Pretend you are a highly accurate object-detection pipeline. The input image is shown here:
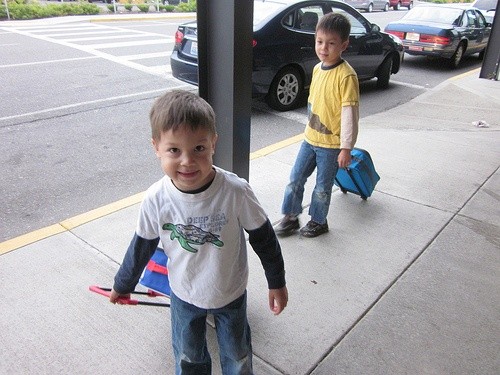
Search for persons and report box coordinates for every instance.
[110,91,289,375]
[273,13,360,238]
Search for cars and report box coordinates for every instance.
[384,2,493,69]
[472,0,497,26]
[345,0,390,13]
[170,0,404,110]
[389,0,414,10]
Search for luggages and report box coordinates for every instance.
[89,248,170,307]
[333,146,380,200]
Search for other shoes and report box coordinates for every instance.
[298,219,328,238]
[273,215,300,235]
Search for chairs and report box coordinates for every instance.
[300,11,318,32]
